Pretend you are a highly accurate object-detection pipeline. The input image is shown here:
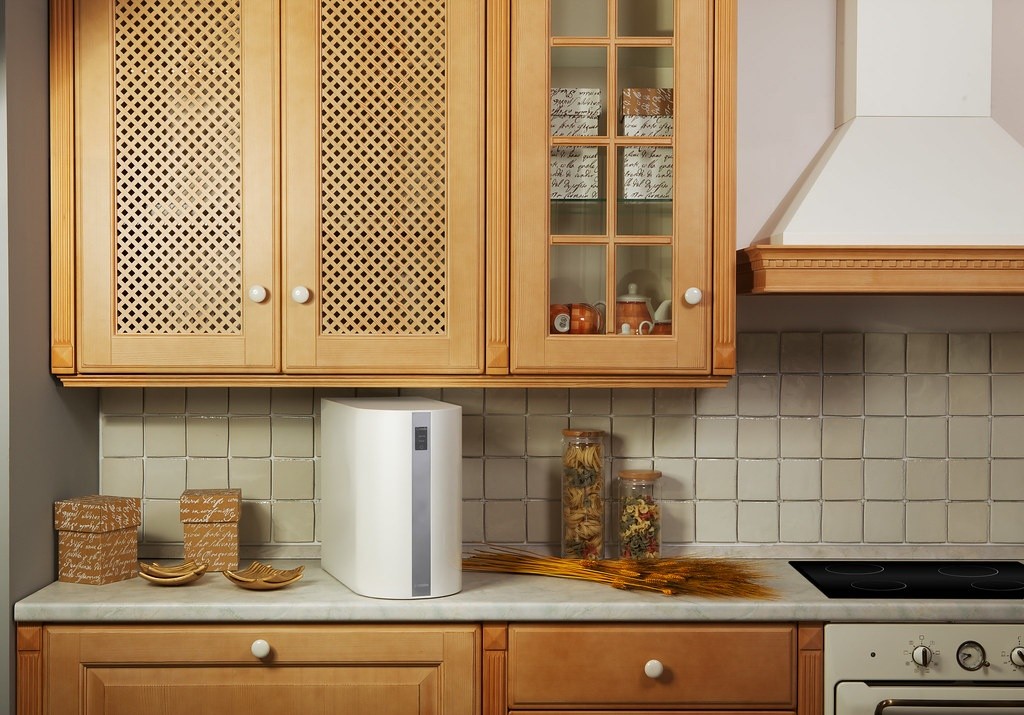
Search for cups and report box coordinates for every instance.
[638,319,672,335]
[551,300,606,334]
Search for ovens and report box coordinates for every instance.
[824,624,1024,715]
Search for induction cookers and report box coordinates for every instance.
[788,560,1024,599]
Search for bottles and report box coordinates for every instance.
[615,469,662,560]
[561,427,606,561]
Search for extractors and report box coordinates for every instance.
[736,0,1023,296]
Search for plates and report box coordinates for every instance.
[222,561,306,590]
[138,557,209,586]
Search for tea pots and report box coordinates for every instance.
[591,283,672,335]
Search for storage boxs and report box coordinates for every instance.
[620,84,676,117]
[549,116,599,199]
[53,493,142,535]
[623,118,674,203]
[185,523,238,575]
[59,532,138,587]
[179,487,241,522]
[547,85,600,115]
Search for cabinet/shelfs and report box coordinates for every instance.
[15,623,824,715]
[51,0,739,389]
